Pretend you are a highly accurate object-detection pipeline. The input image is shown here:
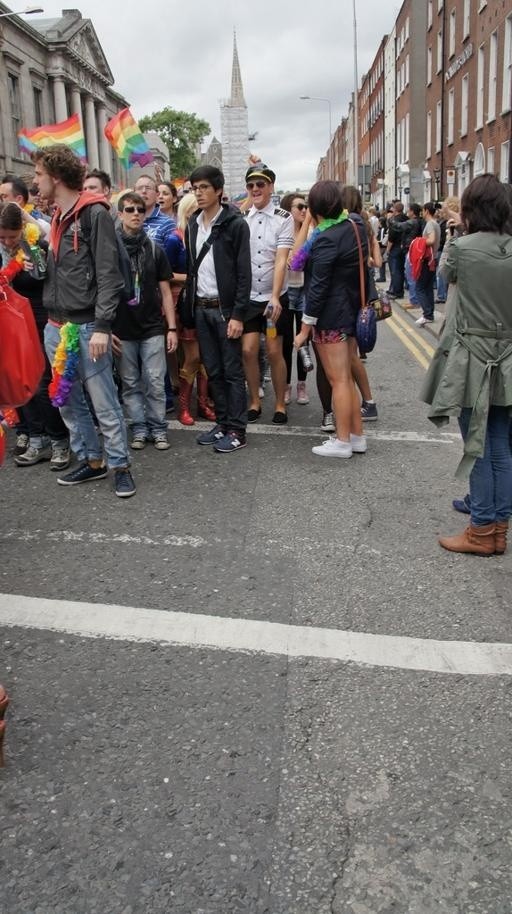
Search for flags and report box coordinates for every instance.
[103,105,154,170]
[17,109,86,167]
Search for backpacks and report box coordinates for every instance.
[78,204,135,302]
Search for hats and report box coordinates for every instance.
[244,162,275,183]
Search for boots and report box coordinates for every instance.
[196,372,217,422]
[439,522,496,558]
[496,517,508,556]
[177,375,196,425]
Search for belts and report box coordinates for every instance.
[195,299,220,310]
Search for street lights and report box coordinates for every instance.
[0,6,44,22]
[301,96,333,181]
[433,167,441,204]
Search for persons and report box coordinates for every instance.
[1,143,385,496]
[362,193,463,326]
[417,174,512,557]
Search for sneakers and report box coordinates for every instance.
[296,382,310,405]
[195,426,227,444]
[114,470,137,498]
[154,433,170,450]
[131,436,147,450]
[272,408,288,424]
[248,375,271,422]
[311,401,377,459]
[416,317,433,324]
[58,462,108,486]
[14,433,70,471]
[214,432,247,452]
[285,384,293,404]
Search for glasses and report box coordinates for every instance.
[191,184,211,192]
[291,204,307,210]
[124,207,145,213]
[246,182,267,189]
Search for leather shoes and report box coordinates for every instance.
[454,500,471,514]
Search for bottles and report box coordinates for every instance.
[28,242,46,278]
[298,341,314,373]
[265,305,278,339]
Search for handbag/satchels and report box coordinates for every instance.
[369,296,393,319]
[174,283,196,330]
[0,284,47,407]
[357,306,378,353]
